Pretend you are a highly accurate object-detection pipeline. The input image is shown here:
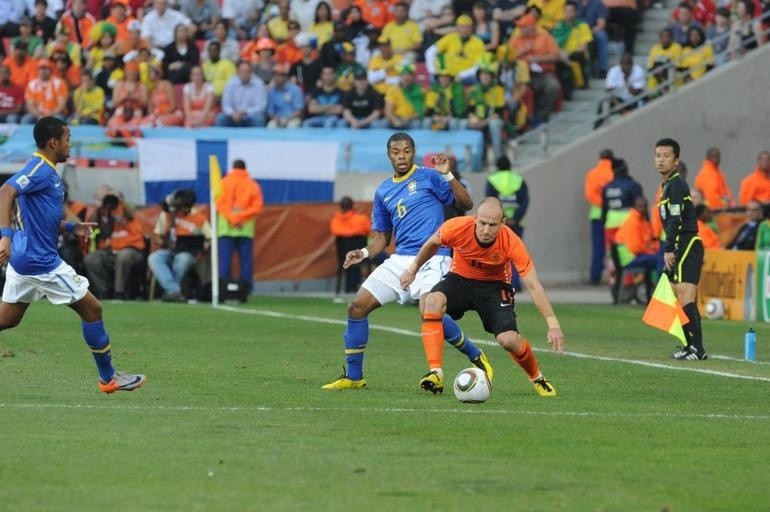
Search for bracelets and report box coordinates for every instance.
[2,226,14,239]
[546,316,561,329]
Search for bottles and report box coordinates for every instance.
[743,327,758,363]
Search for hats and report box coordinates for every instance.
[434,15,537,81]
[333,21,419,73]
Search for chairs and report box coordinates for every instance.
[606,239,653,307]
[334,235,367,296]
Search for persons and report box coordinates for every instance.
[654,139,709,360]
[401,196,564,396]
[59,186,212,302]
[1,116,148,394]
[329,154,529,303]
[0,0,770,141]
[214,160,263,305]
[320,132,493,390]
[584,137,770,303]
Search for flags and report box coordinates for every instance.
[642,274,690,346]
[210,156,223,198]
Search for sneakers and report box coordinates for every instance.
[472,348,493,380]
[680,344,707,360]
[529,371,557,396]
[322,375,367,389]
[671,345,688,359]
[98,373,146,394]
[419,370,443,394]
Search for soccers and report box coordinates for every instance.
[706,299,728,319]
[453,367,492,402]
